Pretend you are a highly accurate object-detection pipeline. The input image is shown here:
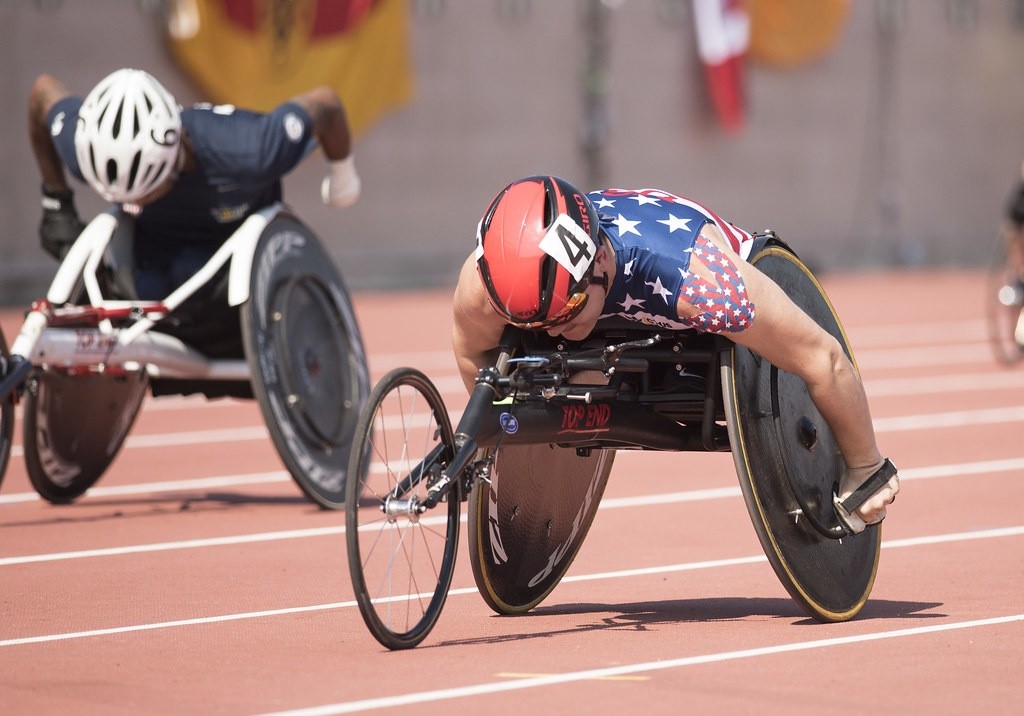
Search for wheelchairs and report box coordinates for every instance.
[344,229,896,650]
[0,203,373,510]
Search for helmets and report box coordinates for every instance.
[74,69,181,204]
[473,175,601,331]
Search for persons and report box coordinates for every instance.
[25,67,366,340]
[454,176,902,527]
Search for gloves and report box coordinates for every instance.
[320,155,361,208]
[34,187,86,259]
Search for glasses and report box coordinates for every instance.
[487,292,590,332]
